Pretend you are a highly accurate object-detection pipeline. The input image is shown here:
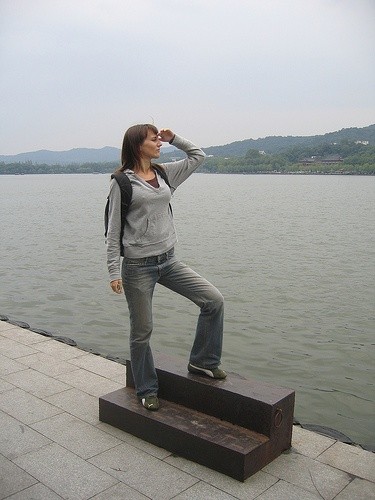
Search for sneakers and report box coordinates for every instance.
[187,362,228,380]
[141,395,160,410]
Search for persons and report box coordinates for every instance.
[105,124,228,411]
